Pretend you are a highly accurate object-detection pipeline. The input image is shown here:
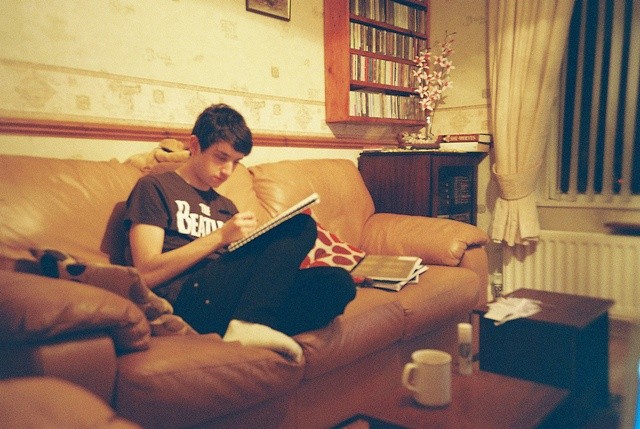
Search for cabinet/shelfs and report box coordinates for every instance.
[471,287,614,407]
[323,0,430,126]
[358,152,488,227]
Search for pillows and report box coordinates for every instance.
[27,245,199,333]
[299,207,369,273]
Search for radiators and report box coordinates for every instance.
[501,229,639,324]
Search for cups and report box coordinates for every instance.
[401,348,452,407]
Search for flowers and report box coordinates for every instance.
[412,29,458,125]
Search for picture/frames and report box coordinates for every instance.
[245,0,292,22]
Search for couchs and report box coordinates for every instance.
[1,153,491,429]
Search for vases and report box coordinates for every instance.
[426,114,434,140]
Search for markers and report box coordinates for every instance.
[458,323,472,375]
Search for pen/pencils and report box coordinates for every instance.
[218,209,234,217]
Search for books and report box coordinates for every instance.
[436,134,493,142]
[228,192,320,252]
[326,2,429,122]
[436,144,489,154]
[352,253,429,292]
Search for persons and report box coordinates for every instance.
[124,103,356,363]
